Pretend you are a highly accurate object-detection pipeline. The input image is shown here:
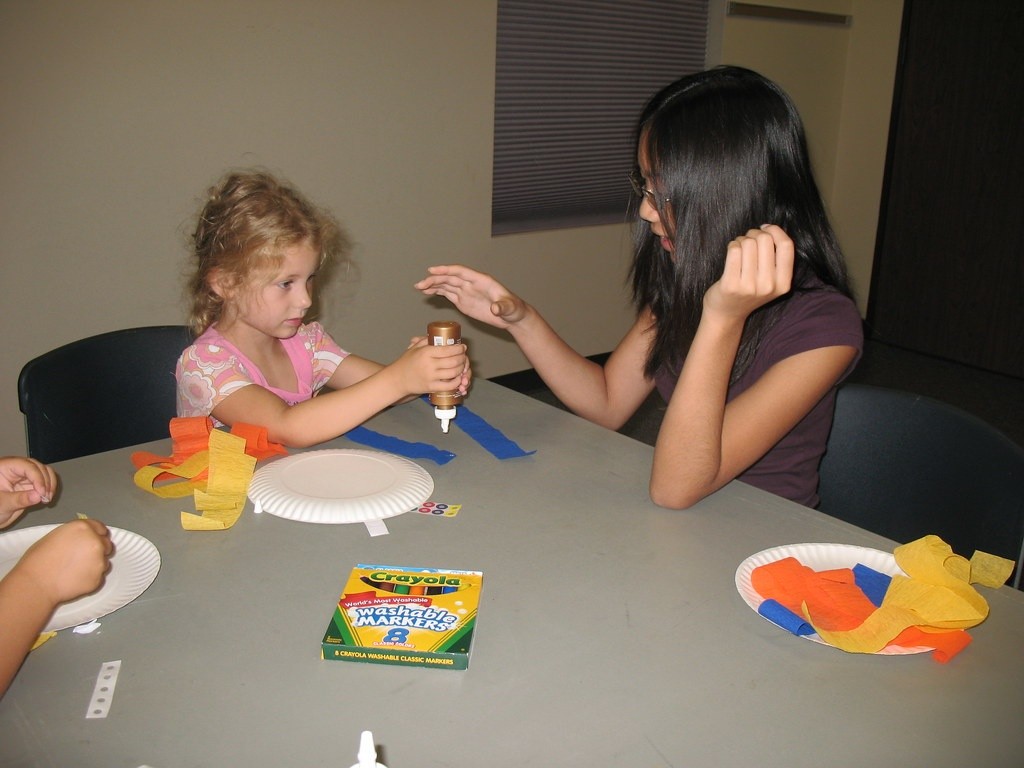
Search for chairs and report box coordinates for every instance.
[18,325,200,463]
[816,383,1023,591]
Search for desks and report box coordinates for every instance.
[0,375,1024,768]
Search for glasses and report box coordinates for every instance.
[627,170,671,211]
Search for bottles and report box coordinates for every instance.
[428,322,461,433]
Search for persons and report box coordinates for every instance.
[0,454,115,703]
[175,170,471,448]
[414,65,865,511]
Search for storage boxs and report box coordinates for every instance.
[320,563,484,670]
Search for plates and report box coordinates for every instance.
[0,523,161,634]
[248,449,435,524]
[735,543,935,655]
